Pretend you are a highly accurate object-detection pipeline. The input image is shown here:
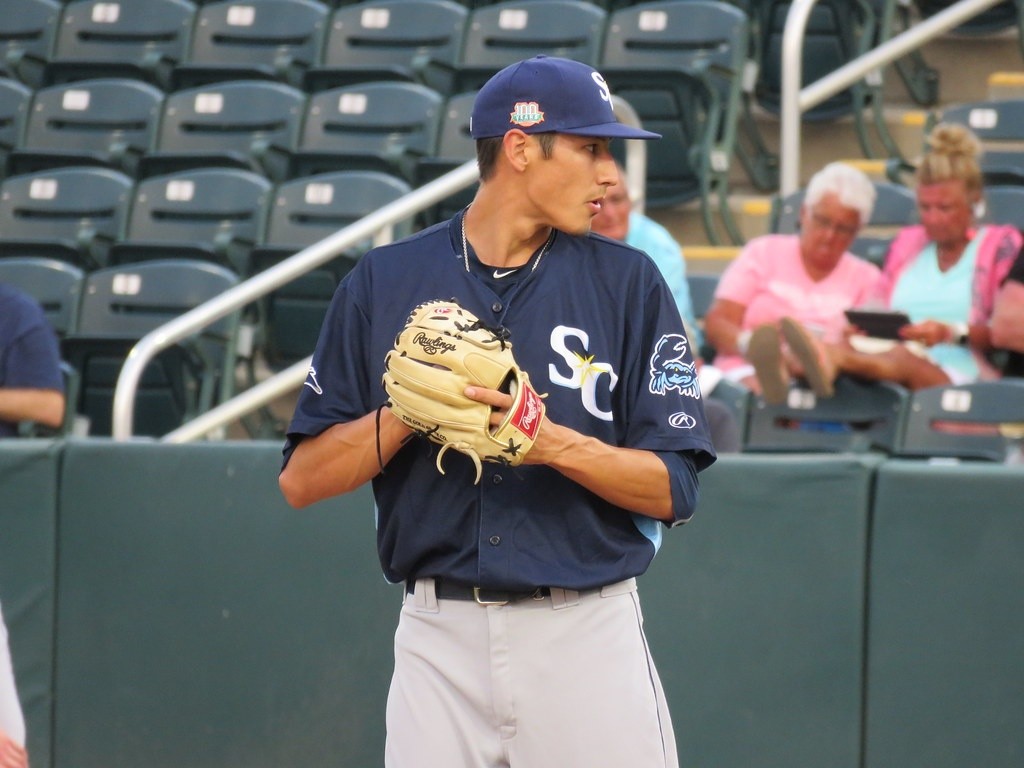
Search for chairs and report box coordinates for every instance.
[0,0,1022,765]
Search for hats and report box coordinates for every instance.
[469,54,663,141]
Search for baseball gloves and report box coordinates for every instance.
[372,295,552,487]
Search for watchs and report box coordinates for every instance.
[955,322,970,346]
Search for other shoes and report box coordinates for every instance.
[778,316,834,401]
[747,324,790,405]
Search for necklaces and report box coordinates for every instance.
[462,214,550,272]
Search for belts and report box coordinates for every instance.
[406,574,551,607]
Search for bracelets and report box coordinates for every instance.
[736,329,753,356]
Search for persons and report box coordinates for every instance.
[0,290,67,768]
[278,52,717,768]
[706,122,1024,439]
[591,162,707,373]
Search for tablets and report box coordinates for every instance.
[842,309,910,340]
